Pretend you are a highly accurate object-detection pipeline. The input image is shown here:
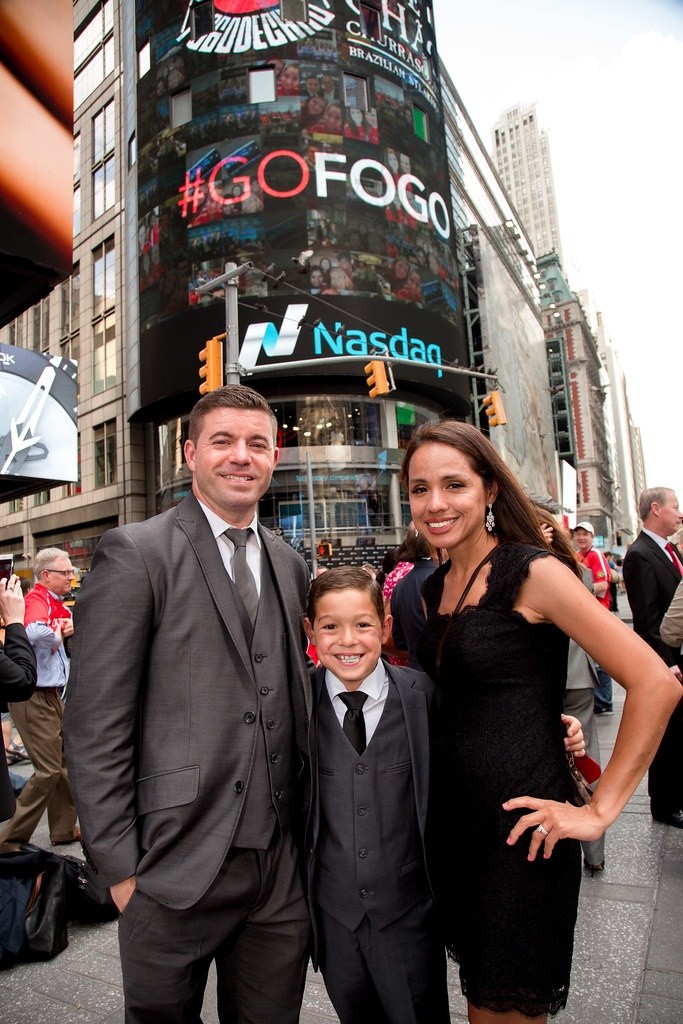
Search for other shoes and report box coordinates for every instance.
[594,704,612,714]
[52,826,81,845]
[653,810,683,829]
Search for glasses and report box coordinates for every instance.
[48,569,74,576]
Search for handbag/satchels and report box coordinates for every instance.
[0,842,119,971]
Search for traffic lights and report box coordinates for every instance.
[199,340,223,396]
[483,391,507,427]
[319,543,332,556]
[364,360,388,398]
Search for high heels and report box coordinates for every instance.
[584,858,604,877]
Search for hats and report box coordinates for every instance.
[573,522,594,535]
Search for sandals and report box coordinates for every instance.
[6,741,30,766]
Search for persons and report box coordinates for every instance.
[137,27,458,312]
[0,547,81,855]
[317,522,622,878]
[401,420,683,1024]
[305,486,586,1024]
[60,384,312,1024]
[622,487,683,828]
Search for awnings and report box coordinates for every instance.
[0,342,78,504]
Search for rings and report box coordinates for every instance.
[537,825,548,835]
[7,586,14,592]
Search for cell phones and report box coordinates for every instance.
[0,553,14,590]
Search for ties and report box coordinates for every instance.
[338,691,368,756]
[664,542,682,577]
[224,530,259,627]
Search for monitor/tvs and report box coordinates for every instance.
[420,280,443,303]
